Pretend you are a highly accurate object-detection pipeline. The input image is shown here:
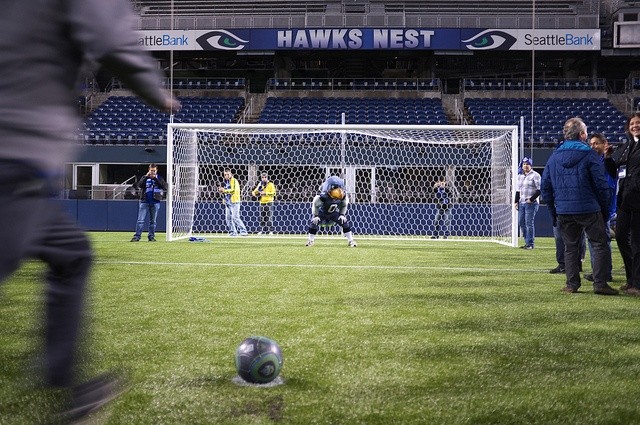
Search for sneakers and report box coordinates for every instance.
[148,238,156,242]
[130,238,139,242]
[584,274,612,282]
[348,240,357,247]
[628,288,640,294]
[620,283,631,290]
[594,284,619,295]
[520,243,534,249]
[305,240,313,246]
[561,286,578,293]
[550,264,565,273]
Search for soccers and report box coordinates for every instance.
[235,337,282,384]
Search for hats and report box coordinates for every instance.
[518,156,533,175]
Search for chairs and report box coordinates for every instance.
[60,93,247,146]
[249,96,452,142]
[465,95,635,148]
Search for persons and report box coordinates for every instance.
[540,118,619,294]
[549,217,586,274]
[0,0,181,419]
[251,169,276,235]
[430,177,451,238]
[305,175,357,247]
[585,134,617,280]
[604,115,640,294]
[129,163,167,242]
[218,168,247,235]
[514,157,542,249]
